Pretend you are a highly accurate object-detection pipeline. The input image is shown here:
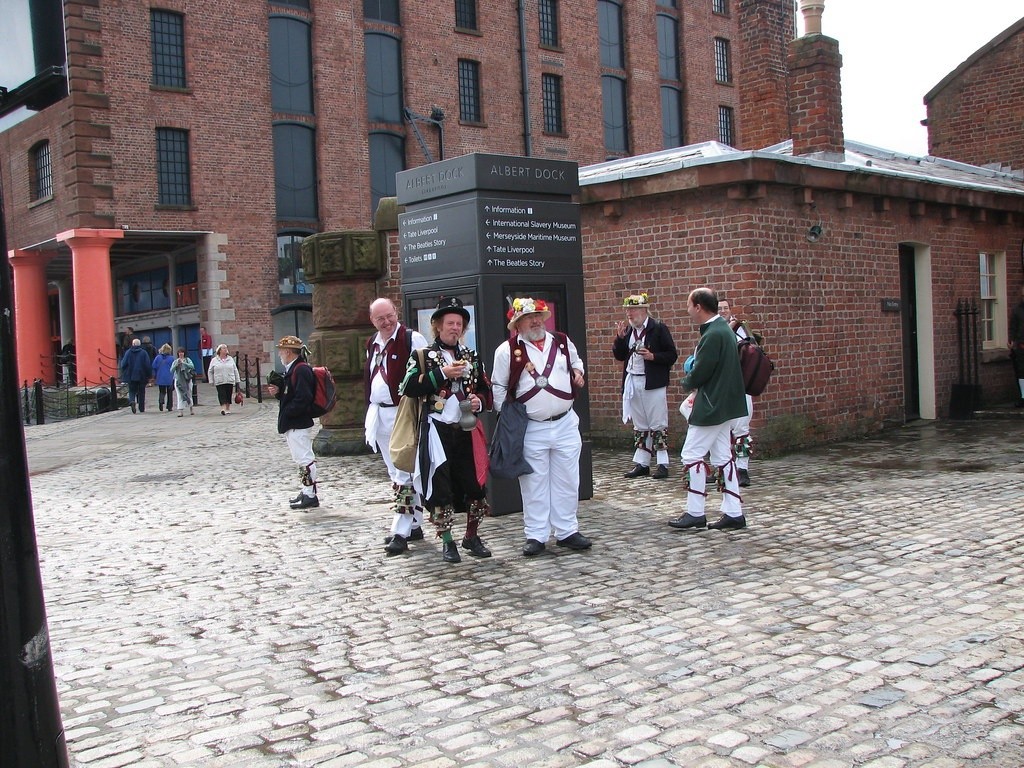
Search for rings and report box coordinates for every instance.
[580,381,583,384]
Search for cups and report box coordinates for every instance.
[636,341,644,354]
[452,360,465,377]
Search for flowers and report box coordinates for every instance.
[506,298,548,318]
[623,293,649,305]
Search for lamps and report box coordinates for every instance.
[805,201,824,242]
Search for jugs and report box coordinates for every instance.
[460,400,477,432]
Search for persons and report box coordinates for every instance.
[121,339,150,413]
[399,298,495,562]
[612,294,677,478]
[170,348,194,417]
[366,300,428,552]
[268,336,320,508]
[200,327,212,383]
[668,288,749,528]
[152,344,174,411]
[706,298,754,485]
[491,299,594,555]
[116,326,157,386]
[63,339,76,386]
[208,344,240,415]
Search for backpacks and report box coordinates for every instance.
[734,332,773,396]
[291,363,339,412]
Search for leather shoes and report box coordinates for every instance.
[706,475,716,483]
[556,533,592,549]
[523,538,545,555]
[384,526,424,544]
[462,534,492,558]
[288,492,319,509]
[652,465,668,478]
[669,512,707,528]
[624,463,650,478]
[384,534,408,554]
[708,513,746,529]
[737,468,750,486]
[442,541,461,562]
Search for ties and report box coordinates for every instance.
[625,326,648,368]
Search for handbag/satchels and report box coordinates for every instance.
[678,389,699,423]
[235,390,244,404]
[390,395,419,474]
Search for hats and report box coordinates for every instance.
[506,294,551,332]
[431,297,471,328]
[622,295,650,308]
[273,335,304,349]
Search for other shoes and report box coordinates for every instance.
[130,400,231,417]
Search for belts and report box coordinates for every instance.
[379,402,397,408]
[632,373,645,377]
[527,407,572,421]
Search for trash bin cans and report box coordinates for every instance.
[97,387,111,413]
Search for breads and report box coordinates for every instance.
[452,360,467,367]
